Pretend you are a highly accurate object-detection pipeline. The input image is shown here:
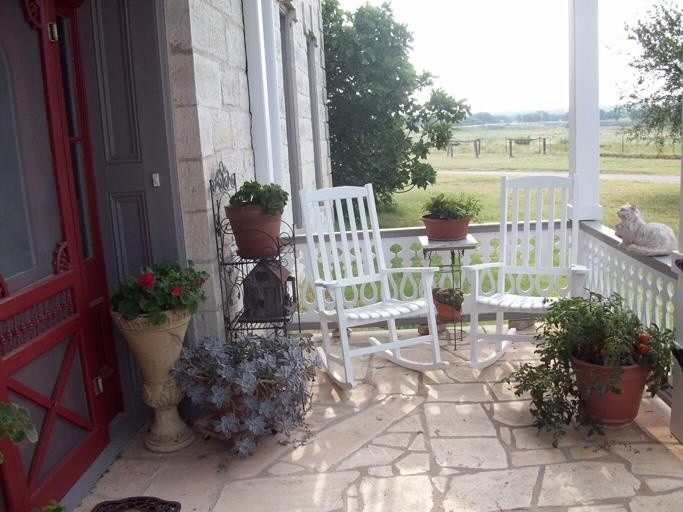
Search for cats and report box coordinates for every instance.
[614,202,679,256]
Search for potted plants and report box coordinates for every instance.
[493,283,677,454]
[418,192,482,240]
[222,179,289,258]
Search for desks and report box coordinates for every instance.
[418,232,481,350]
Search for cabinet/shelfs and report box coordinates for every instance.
[209,161,302,339]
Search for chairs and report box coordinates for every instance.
[297,181,450,391]
[459,170,589,369]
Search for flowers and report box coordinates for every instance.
[168,332,324,460]
[97,254,211,325]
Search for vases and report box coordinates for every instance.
[109,305,197,454]
